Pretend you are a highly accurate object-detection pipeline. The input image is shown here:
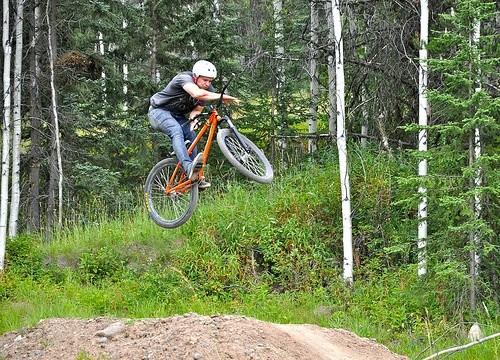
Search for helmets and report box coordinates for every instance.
[193,60,217,79]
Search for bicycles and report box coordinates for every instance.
[144,73,274,228]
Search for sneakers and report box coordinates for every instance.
[198,180,211,188]
[189,153,204,181]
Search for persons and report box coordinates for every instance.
[147,59,241,189]
